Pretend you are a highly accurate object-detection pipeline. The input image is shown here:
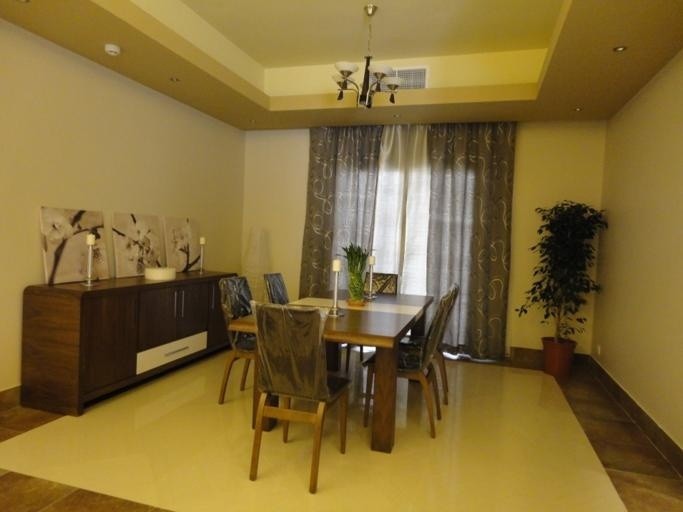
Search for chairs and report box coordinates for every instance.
[344,272,401,374]
[261,272,288,304]
[220,276,264,405]
[363,283,460,439]
[247,300,355,494]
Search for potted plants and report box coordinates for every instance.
[511,201,607,376]
[339,242,379,309]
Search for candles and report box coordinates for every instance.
[329,255,376,271]
[200,237,206,244]
[86,233,96,245]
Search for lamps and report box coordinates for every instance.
[330,3,399,109]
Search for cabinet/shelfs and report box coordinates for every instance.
[18,267,239,419]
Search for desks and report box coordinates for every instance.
[226,288,435,457]
